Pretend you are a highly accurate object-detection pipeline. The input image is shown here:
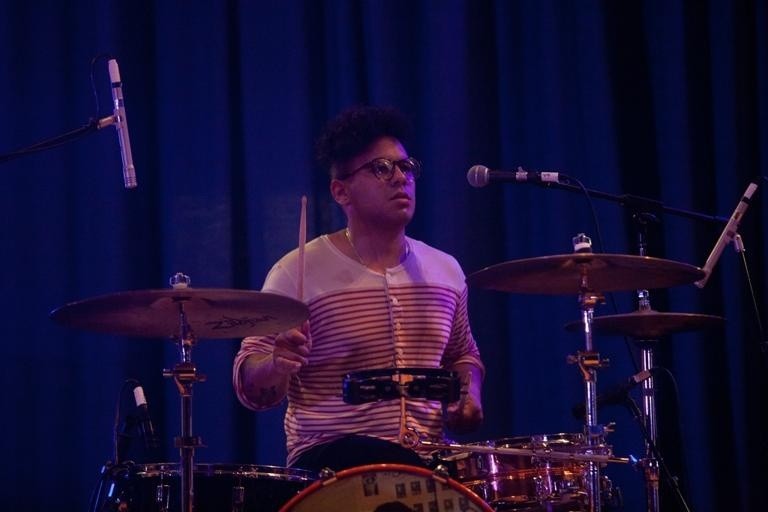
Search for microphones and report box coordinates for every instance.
[694,183,757,288]
[108,60,138,188]
[572,369,653,419]
[132,382,156,443]
[467,165,569,187]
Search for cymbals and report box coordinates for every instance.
[465,252,706,293]
[50,289,310,340]
[562,311,727,338]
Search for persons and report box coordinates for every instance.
[231,105,485,475]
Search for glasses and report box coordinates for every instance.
[337,156,421,183]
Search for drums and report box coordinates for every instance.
[428,433,615,511]
[278,463,494,512]
[126,462,318,512]
